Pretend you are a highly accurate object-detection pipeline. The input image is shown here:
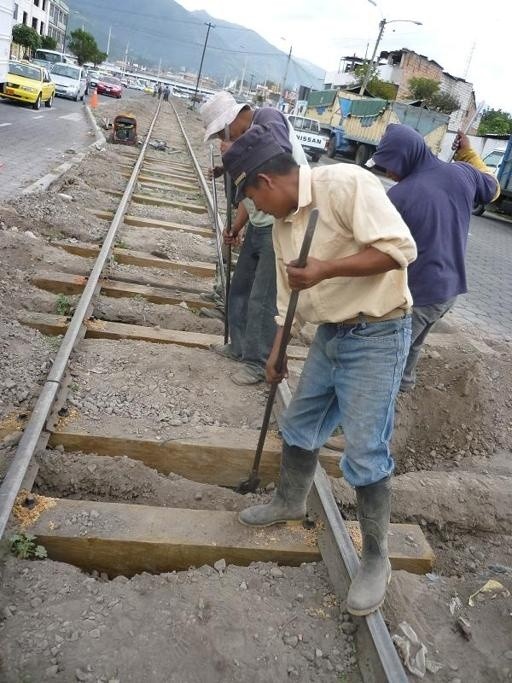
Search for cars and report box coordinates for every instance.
[31,47,154,101]
[0,59,56,109]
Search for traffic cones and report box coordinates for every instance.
[90,86,98,107]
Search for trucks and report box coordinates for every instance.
[303,88,512,221]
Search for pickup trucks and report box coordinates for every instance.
[283,113,329,163]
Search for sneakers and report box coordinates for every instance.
[200,306,224,320]
[324,433,345,450]
[231,369,260,385]
[201,292,220,302]
[210,343,241,361]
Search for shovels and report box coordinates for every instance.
[237,208,319,495]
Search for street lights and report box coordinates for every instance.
[357,0,427,95]
[279,35,295,110]
[238,44,248,93]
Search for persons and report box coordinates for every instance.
[194,87,312,386]
[222,121,418,616]
[199,163,239,322]
[153,83,172,102]
[372,121,503,395]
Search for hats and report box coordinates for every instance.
[199,89,251,143]
[222,124,284,203]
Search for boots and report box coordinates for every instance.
[346,476,391,617]
[237,441,320,528]
